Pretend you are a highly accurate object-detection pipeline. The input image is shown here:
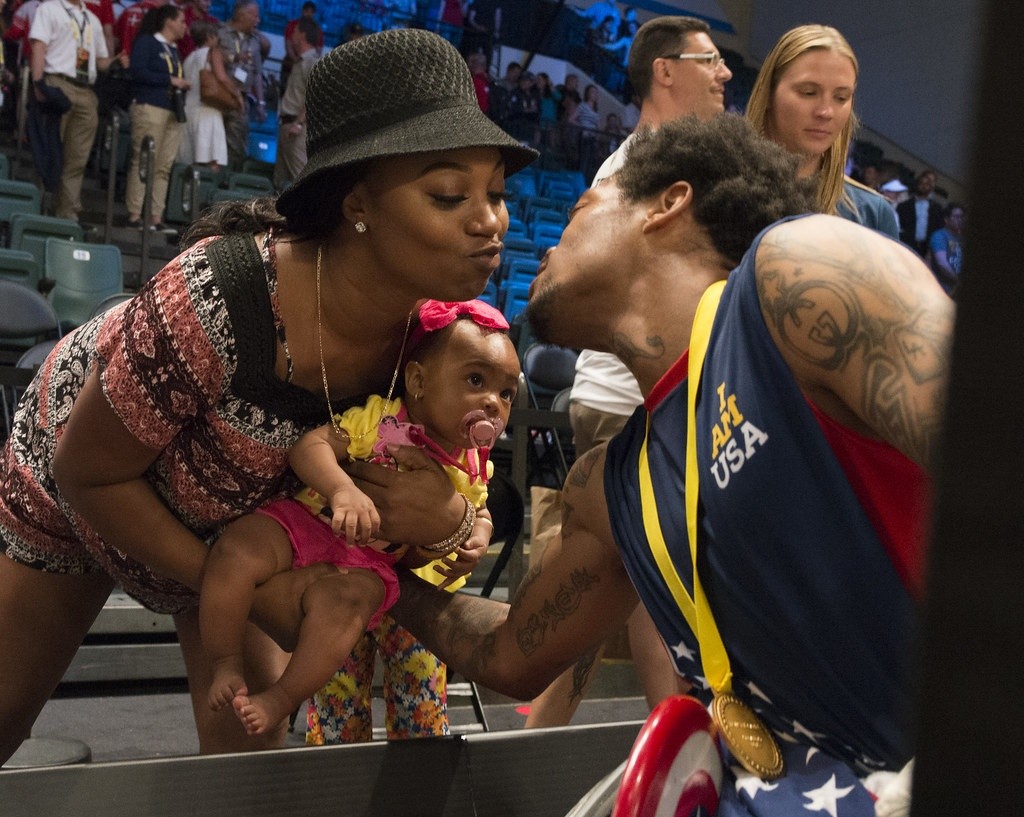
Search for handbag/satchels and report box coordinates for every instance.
[199,69,240,110]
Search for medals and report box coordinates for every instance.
[712,692,784,779]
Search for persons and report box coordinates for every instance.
[440,0,639,181]
[199,300,520,735]
[523,18,732,728]
[0,30,538,762]
[0,0,327,233]
[747,24,898,238]
[844,141,962,297]
[306,613,450,744]
[390,111,953,817]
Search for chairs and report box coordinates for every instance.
[0,101,586,773]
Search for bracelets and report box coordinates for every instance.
[295,122,303,127]
[258,101,266,105]
[421,493,477,553]
[477,516,495,537]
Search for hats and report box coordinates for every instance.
[276,28,540,233]
[878,179,909,193]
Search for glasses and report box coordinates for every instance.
[661,51,725,72]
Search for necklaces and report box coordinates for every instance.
[316,248,412,440]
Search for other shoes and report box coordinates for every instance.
[76,219,97,234]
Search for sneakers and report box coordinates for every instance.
[125,218,156,231]
[154,222,178,234]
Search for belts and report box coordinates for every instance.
[44,72,92,89]
[278,115,295,125]
